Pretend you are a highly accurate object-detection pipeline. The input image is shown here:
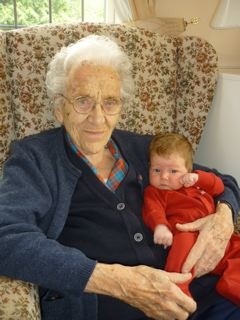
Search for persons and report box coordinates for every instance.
[142,133,240,303]
[0,35,240,320]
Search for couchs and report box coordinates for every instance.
[0,20,218,320]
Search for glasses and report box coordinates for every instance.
[60,93,124,114]
